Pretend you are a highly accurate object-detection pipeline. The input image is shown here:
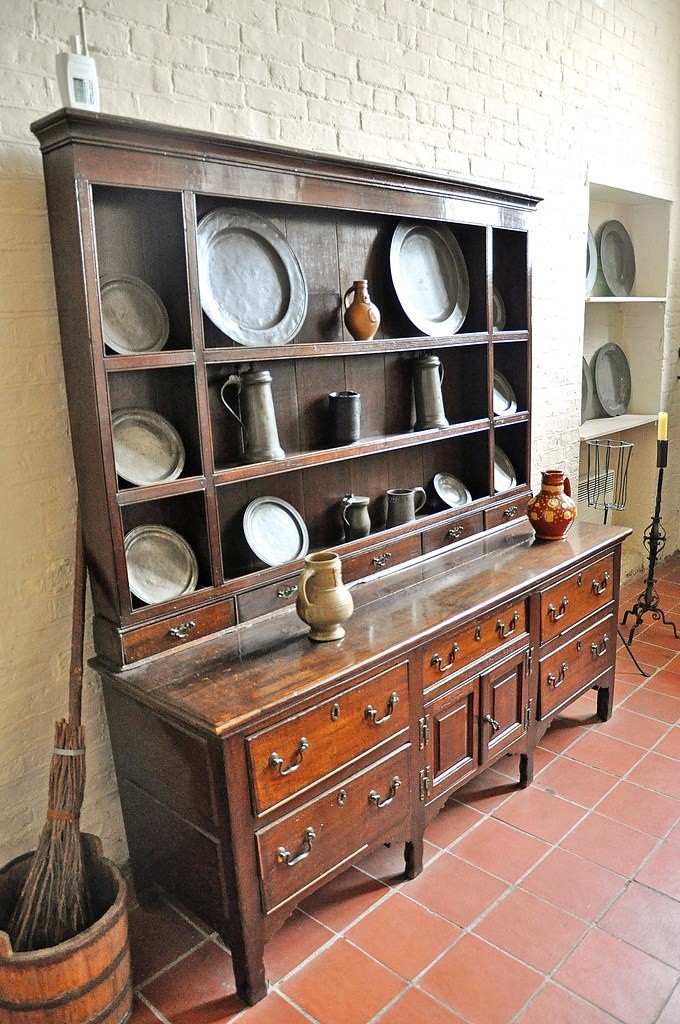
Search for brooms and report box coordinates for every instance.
[5,494,93,953]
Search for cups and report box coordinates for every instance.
[342,494,370,543]
[385,487,427,529]
[328,391,361,445]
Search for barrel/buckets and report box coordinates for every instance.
[1,833,133,1024]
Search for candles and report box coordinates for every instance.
[657,412,668,440]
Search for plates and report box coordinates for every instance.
[494,445,517,492]
[494,284,507,331]
[493,368,518,414]
[582,221,635,424]
[433,471,472,508]
[123,523,200,608]
[98,274,170,355]
[197,207,308,345]
[243,495,310,568]
[389,216,470,336]
[111,407,186,487]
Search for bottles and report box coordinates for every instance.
[525,471,576,541]
[296,552,354,642]
[344,281,380,341]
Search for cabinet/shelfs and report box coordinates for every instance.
[31,105,665,1007]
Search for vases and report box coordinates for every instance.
[344,280,380,341]
[297,552,354,641]
[528,470,577,540]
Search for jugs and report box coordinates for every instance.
[221,362,286,465]
[412,350,450,431]
